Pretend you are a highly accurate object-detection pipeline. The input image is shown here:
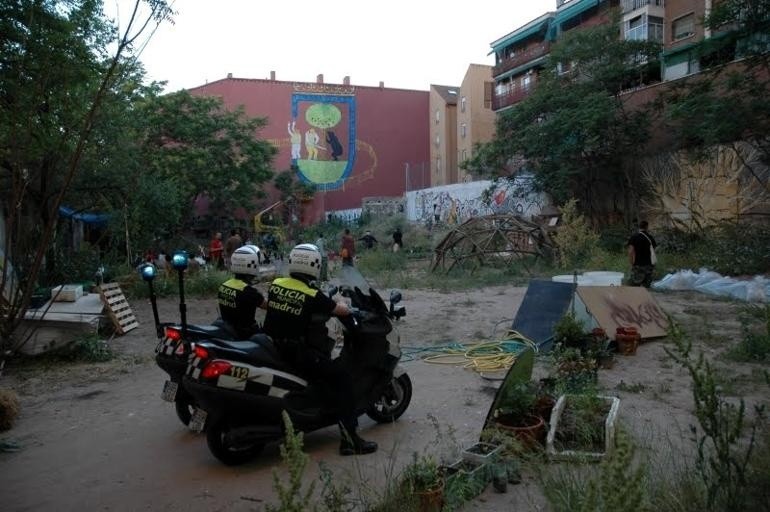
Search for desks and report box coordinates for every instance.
[14,290,116,351]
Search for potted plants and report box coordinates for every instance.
[549,324,620,462]
[399,413,522,512]
[492,378,555,458]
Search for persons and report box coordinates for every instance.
[338,229,356,266]
[224,229,240,271]
[358,230,377,249]
[210,231,226,270]
[392,228,404,249]
[627,220,658,287]
[266,243,381,454]
[316,233,333,281]
[218,246,268,335]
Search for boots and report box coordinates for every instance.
[340,431,377,456]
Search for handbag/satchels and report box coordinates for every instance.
[340,248,348,258]
[650,244,657,265]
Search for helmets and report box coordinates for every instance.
[231,245,265,276]
[289,243,322,279]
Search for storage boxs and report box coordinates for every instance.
[51,282,83,301]
